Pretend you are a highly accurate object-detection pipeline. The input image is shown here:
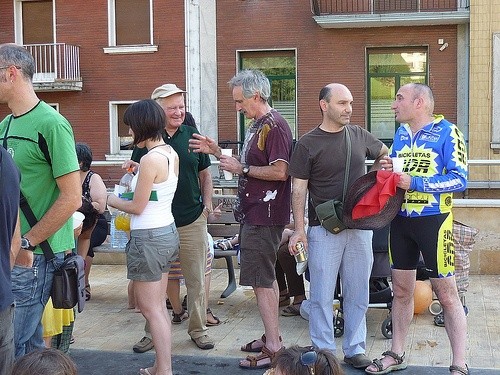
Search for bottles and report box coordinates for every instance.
[115,168,135,229]
[110,214,128,249]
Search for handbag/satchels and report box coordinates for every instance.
[51,254,85,313]
[314,199,344,234]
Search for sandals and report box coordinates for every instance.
[85,283,91,302]
[449,364,469,375]
[217,241,233,251]
[365,351,407,375]
[172,309,189,324]
[205,308,221,326]
[238,346,285,369]
[140,367,151,375]
[240,334,282,351]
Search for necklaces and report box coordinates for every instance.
[148,144,167,152]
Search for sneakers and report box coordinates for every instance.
[190,334,214,350]
[435,307,468,328]
[344,353,372,368]
[132,336,155,353]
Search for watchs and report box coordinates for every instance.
[21,235,36,252]
[242,164,250,180]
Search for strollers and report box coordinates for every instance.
[332,224,395,340]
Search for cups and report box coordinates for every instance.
[392,157,403,172]
[221,149,233,180]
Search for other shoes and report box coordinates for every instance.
[166,298,173,310]
[70,336,75,344]
[182,294,188,310]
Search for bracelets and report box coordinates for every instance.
[204,206,212,213]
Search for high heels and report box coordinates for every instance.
[279,293,291,307]
[280,297,307,316]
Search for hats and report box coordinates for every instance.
[151,83,187,100]
[342,170,406,230]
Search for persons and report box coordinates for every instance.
[365,82,467,375]
[0,43,109,362]
[12,347,78,375]
[0,144,22,375]
[218,139,309,316]
[129,83,222,353]
[288,83,390,368]
[189,70,293,368]
[106,98,182,375]
[271,344,342,375]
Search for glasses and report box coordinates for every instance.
[300,346,317,375]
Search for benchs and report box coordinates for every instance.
[204,196,241,297]
